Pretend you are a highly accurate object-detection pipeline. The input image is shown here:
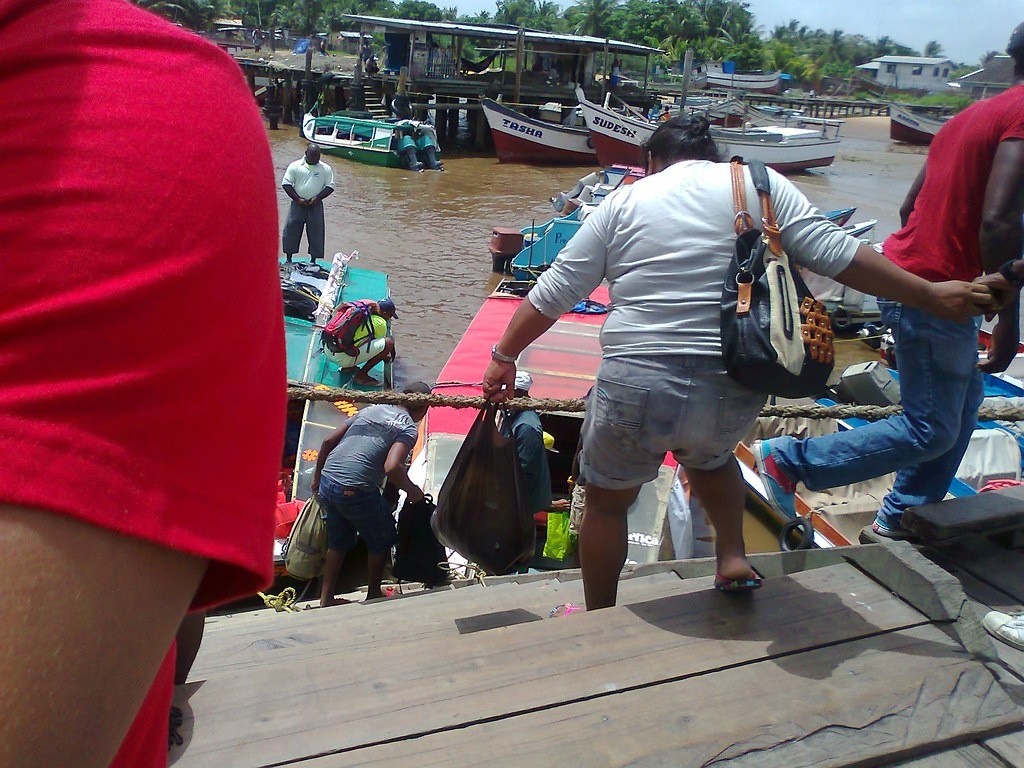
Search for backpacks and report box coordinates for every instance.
[321,299,377,357]
[281,491,328,580]
[392,493,451,585]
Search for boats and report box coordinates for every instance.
[889,100,943,143]
[408,161,705,576]
[576,87,838,175]
[303,109,445,174]
[202,255,393,619]
[735,206,1024,648]
[478,90,599,164]
[705,61,781,95]
[487,163,877,306]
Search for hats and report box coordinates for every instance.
[403,380,431,395]
[377,299,399,319]
[515,371,534,391]
[543,431,558,452]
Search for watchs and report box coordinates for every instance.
[999,259,1024,291]
[491,345,519,363]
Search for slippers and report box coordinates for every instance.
[714,566,764,591]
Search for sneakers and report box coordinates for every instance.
[872,516,916,537]
[982,611,1024,652]
[753,440,796,523]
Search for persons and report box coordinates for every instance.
[0,1,286,768]
[230,24,1024,619]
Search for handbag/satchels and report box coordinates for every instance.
[430,397,536,575]
[543,511,576,562]
[719,228,835,400]
